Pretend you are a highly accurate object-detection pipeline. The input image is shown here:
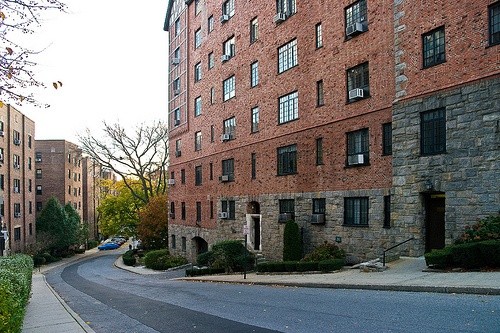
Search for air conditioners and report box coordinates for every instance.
[170,58,180,65]
[218,211,228,220]
[349,88,363,100]
[218,175,228,183]
[347,22,362,36]
[309,213,324,224]
[0,131,5,136]
[173,90,179,96]
[220,134,230,141]
[347,154,364,166]
[275,12,286,23]
[167,179,175,186]
[219,14,229,23]
[173,119,179,126]
[278,213,291,224]
[14,140,21,145]
[13,187,18,193]
[15,212,21,217]
[220,55,229,63]
[169,213,174,218]
[0,154,4,160]
[174,151,181,157]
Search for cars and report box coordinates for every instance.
[97,234,128,250]
